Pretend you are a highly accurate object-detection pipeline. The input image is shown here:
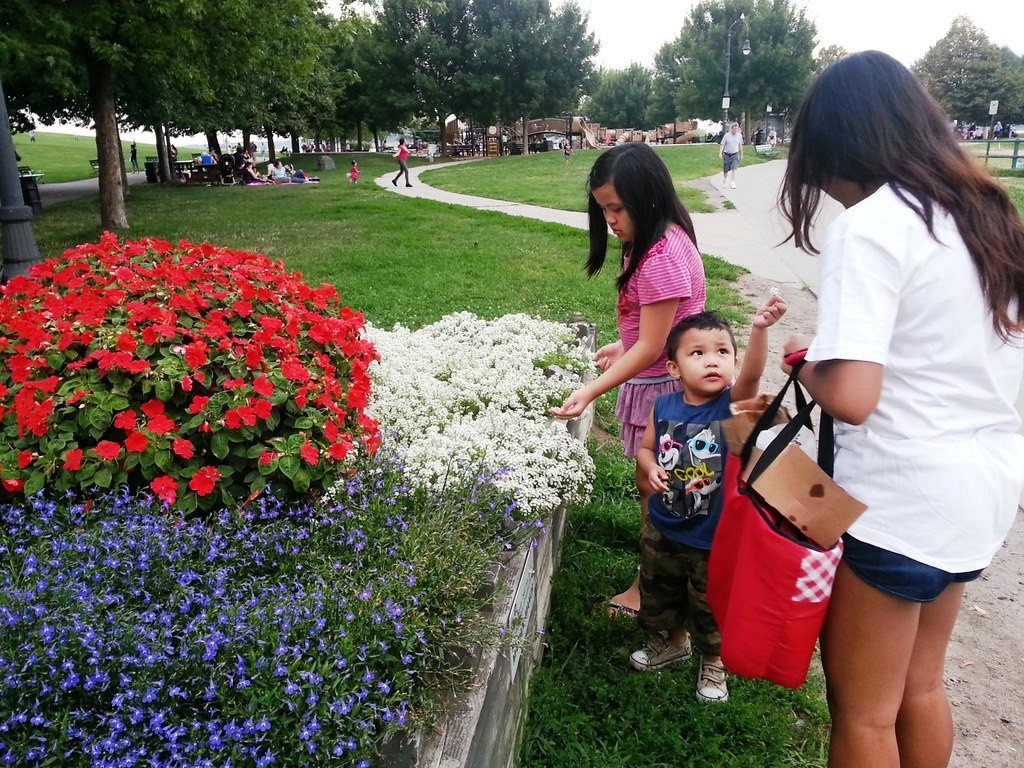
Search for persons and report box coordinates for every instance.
[953,119,1005,141]
[392,137,414,187]
[628,293,788,704]
[718,121,744,189]
[771,49,1023,768]
[170,141,331,184]
[382,138,423,156]
[509,135,546,153]
[547,141,707,617]
[129,145,139,174]
[707,122,777,149]
[349,159,362,185]
[563,139,574,167]
[30,131,36,142]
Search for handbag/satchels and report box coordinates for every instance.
[704,347,845,689]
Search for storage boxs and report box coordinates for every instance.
[202,156,213,165]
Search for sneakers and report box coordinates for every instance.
[629,634,693,671]
[695,654,730,704]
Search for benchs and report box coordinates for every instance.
[755,143,781,160]
[89,159,99,174]
[17,165,45,184]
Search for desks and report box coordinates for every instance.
[194,163,221,186]
[176,160,193,178]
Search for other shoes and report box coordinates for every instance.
[732,180,736,188]
[392,180,397,186]
[406,183,412,187]
[723,179,728,188]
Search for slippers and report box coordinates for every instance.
[590,599,641,622]
